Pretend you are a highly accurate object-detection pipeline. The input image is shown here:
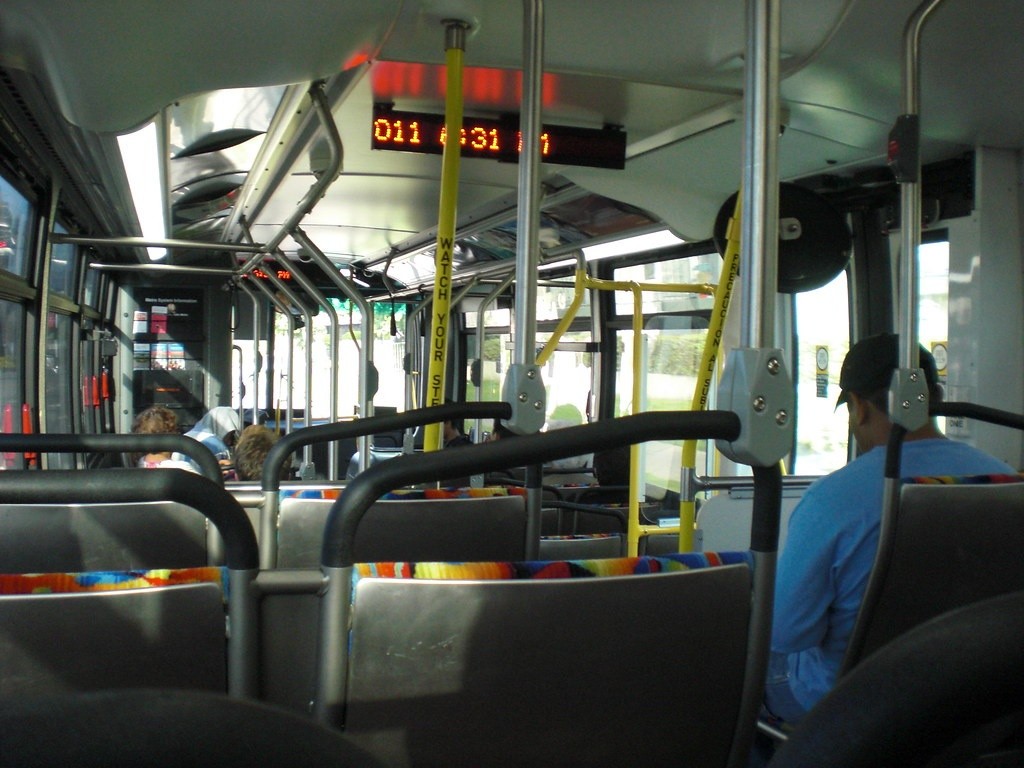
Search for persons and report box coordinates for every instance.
[171,406,239,461]
[131,406,200,475]
[441,398,473,487]
[233,426,292,481]
[749,333,1018,767]
[484,417,524,487]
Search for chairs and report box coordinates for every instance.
[0,402,1024,768]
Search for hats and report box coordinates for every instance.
[834,333,937,412]
